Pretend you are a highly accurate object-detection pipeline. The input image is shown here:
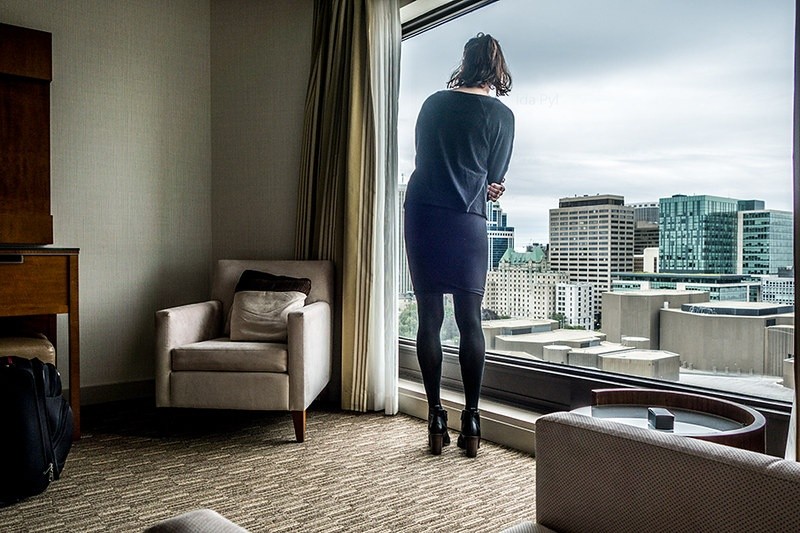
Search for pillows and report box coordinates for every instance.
[226,270,311,311]
[224,290,309,341]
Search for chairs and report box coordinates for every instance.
[154,259,334,444]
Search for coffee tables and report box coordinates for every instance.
[570,388,766,454]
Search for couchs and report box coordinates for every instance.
[143,412,800,533]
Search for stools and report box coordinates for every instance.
[0,334,56,367]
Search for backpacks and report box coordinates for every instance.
[0,354,75,504]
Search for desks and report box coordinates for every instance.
[0,248,81,439]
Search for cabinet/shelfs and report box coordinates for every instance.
[0,22,55,246]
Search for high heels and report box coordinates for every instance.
[427,405,451,455]
[457,409,482,458]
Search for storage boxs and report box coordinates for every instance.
[647,408,674,430]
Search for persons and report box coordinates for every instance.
[403,33,515,458]
